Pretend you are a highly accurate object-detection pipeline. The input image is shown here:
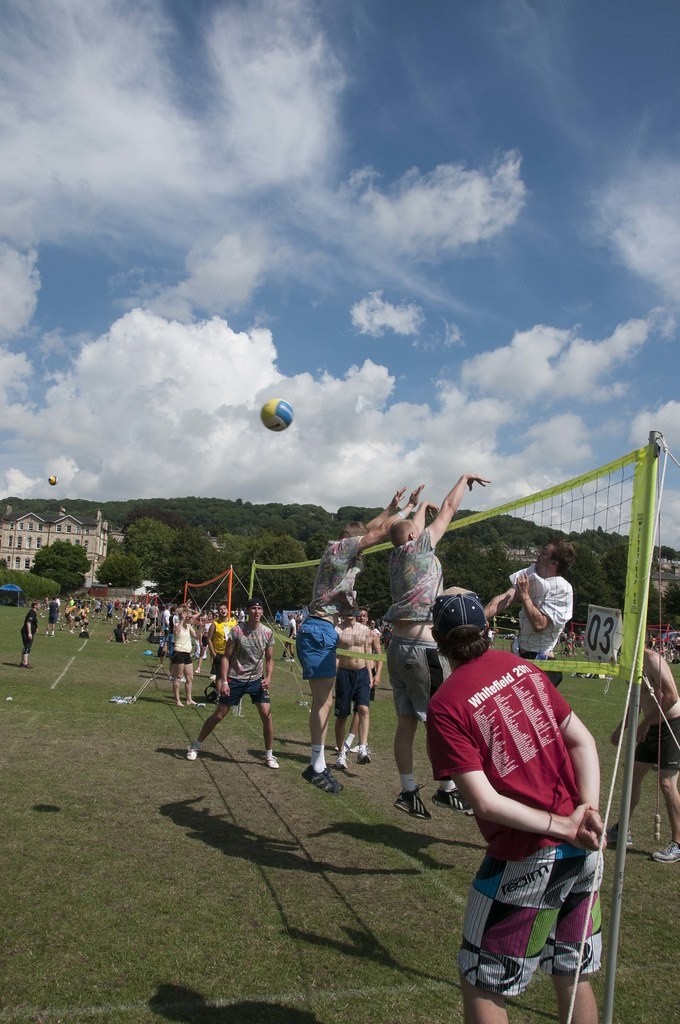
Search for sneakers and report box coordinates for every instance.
[432,787,475,816]
[302,764,343,794]
[394,783,431,819]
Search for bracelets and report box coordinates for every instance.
[222,682,228,685]
[397,511,408,520]
[418,505,427,511]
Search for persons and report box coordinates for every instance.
[21,583,246,708]
[645,629,680,664]
[279,595,589,666]
[186,599,280,769]
[611,648,680,864]
[382,472,492,822]
[296,484,426,794]
[334,605,382,771]
[426,587,607,1024]
[483,538,573,689]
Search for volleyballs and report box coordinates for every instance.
[48,476,58,485]
[261,398,294,432]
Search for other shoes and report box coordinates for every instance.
[266,755,279,768]
[350,746,371,753]
[606,825,632,845]
[20,662,32,668]
[356,750,371,765]
[334,742,348,753]
[652,840,680,863]
[336,756,347,768]
[187,742,198,760]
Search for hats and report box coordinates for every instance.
[433,586,486,646]
[246,598,263,609]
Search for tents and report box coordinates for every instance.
[0,584,23,608]
